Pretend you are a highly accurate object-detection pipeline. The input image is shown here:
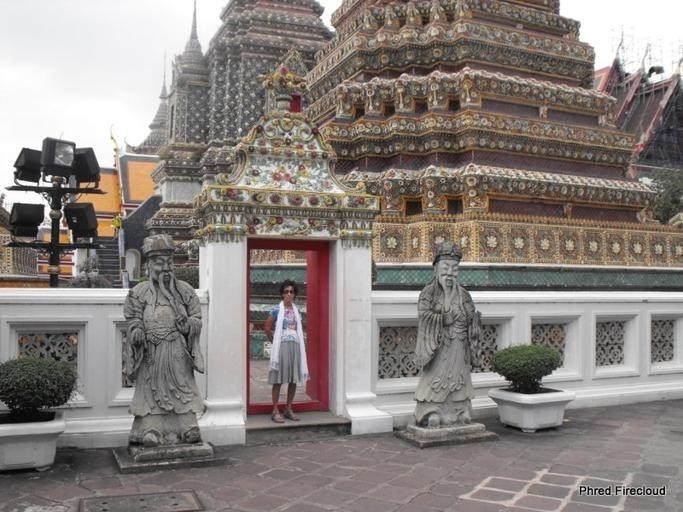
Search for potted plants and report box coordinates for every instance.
[487,343,576,434]
[0,355,78,472]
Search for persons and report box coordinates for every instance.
[409,242,484,429]
[262,278,310,424]
[119,232,207,449]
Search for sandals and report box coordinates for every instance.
[272,410,300,423]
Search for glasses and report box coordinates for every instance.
[283,290,295,294]
